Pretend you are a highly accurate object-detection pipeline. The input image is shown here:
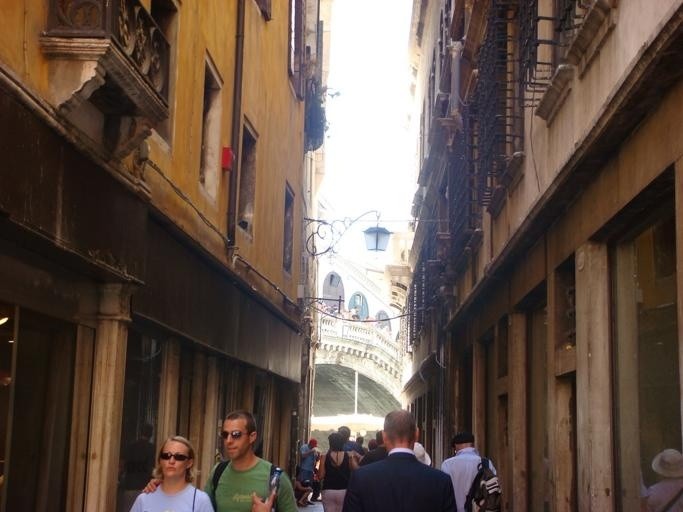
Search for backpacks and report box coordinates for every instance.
[463,456,502,512]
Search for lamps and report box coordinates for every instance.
[303,209,393,259]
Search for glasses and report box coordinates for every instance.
[158,451,190,461]
[219,429,250,439]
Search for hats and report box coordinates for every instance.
[309,438,317,448]
[452,433,474,447]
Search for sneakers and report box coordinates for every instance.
[296,496,321,507]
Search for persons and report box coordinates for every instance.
[130,435,214,512]
[119,422,155,490]
[640,448,683,512]
[349,305,361,322]
[441,433,496,512]
[144,410,300,512]
[319,411,457,512]
[296,439,321,506]
